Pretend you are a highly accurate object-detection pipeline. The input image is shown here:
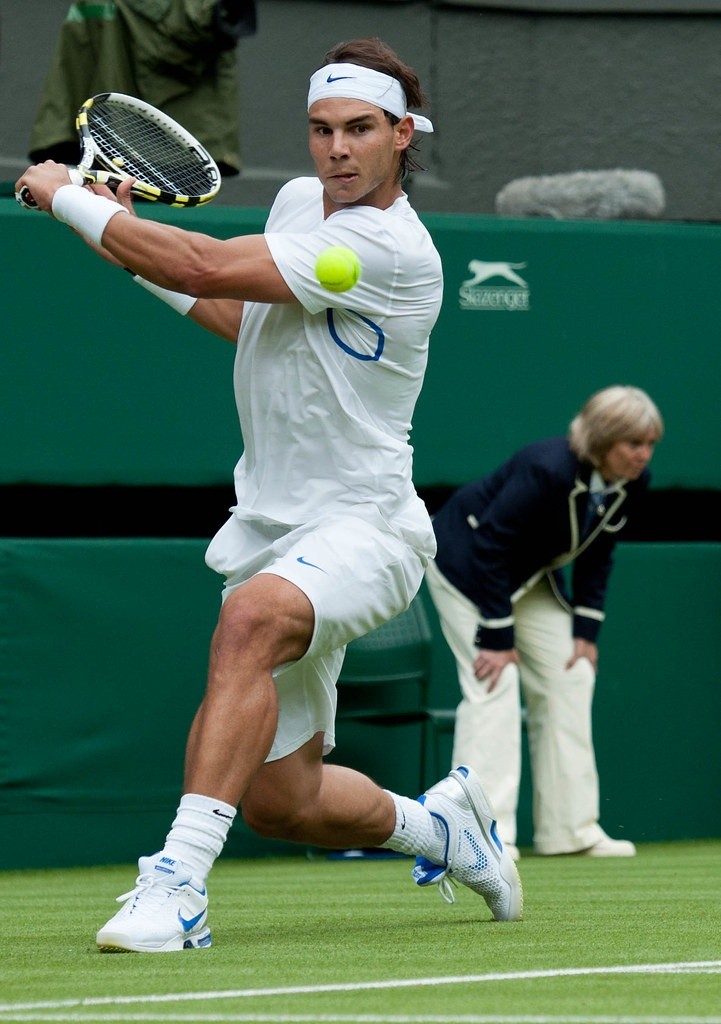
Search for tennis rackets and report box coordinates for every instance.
[18,90,223,212]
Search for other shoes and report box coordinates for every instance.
[578,836,637,860]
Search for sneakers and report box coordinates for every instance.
[95,849,212,954]
[408,772,525,923]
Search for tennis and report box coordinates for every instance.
[316,247,361,293]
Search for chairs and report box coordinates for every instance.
[336,593,530,797]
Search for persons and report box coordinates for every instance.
[16,38,524,952]
[425,385,664,859]
